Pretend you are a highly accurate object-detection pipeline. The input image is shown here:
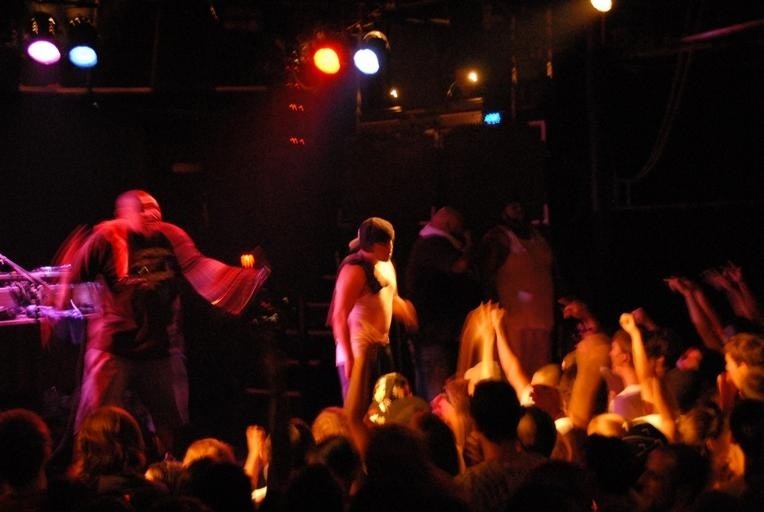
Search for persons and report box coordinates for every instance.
[0,189,764,512]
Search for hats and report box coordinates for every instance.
[348,217,395,249]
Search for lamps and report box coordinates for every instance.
[63,16,105,78]
[20,11,64,69]
[312,30,348,78]
[348,22,394,78]
